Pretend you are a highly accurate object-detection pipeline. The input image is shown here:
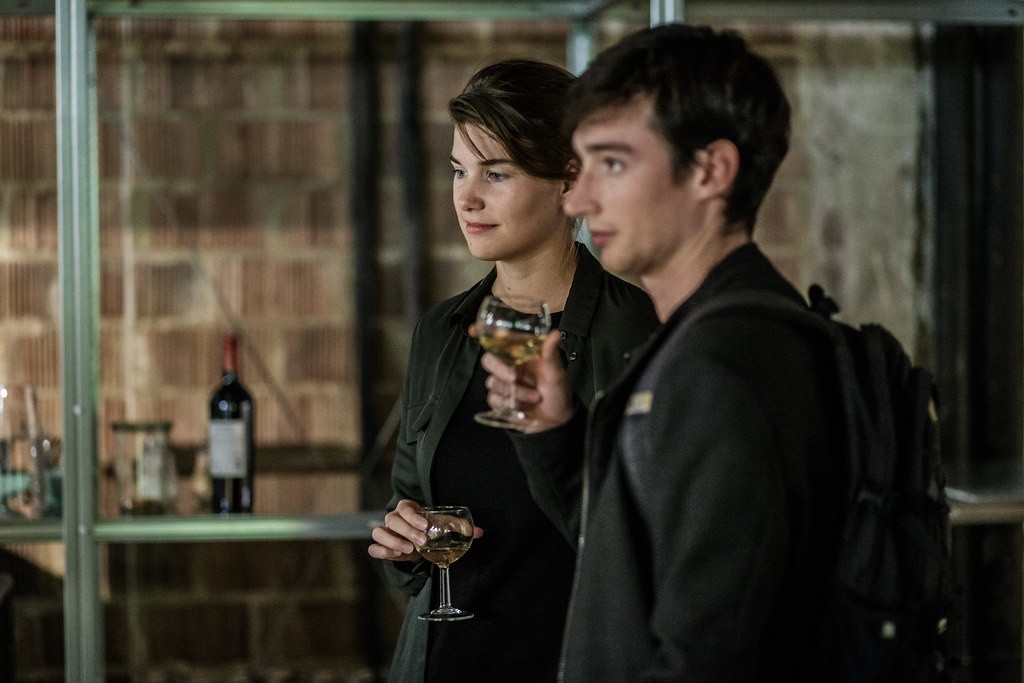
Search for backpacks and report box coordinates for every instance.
[623,283,947,683]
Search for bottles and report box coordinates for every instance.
[207,334,257,514]
[1,382,49,525]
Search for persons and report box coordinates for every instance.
[469,21,847,683]
[369,57,665,683]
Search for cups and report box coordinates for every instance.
[110,420,172,516]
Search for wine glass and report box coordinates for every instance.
[414,506,474,622]
[473,294,551,430]
